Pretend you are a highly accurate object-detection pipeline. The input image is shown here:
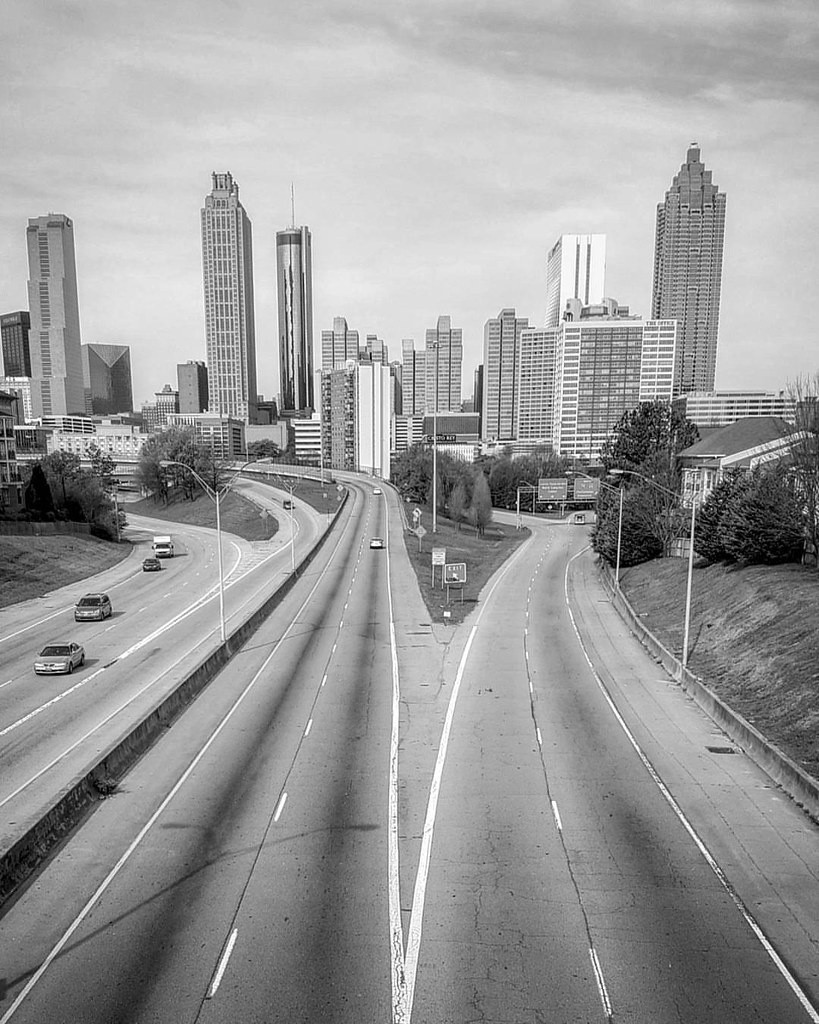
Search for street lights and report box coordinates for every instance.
[564,469,625,583]
[609,468,697,667]
[159,456,273,642]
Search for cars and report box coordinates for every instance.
[369,537,384,549]
[33,641,86,676]
[282,499,294,510]
[373,487,381,495]
[74,593,113,624]
[152,543,176,560]
[142,558,161,571]
